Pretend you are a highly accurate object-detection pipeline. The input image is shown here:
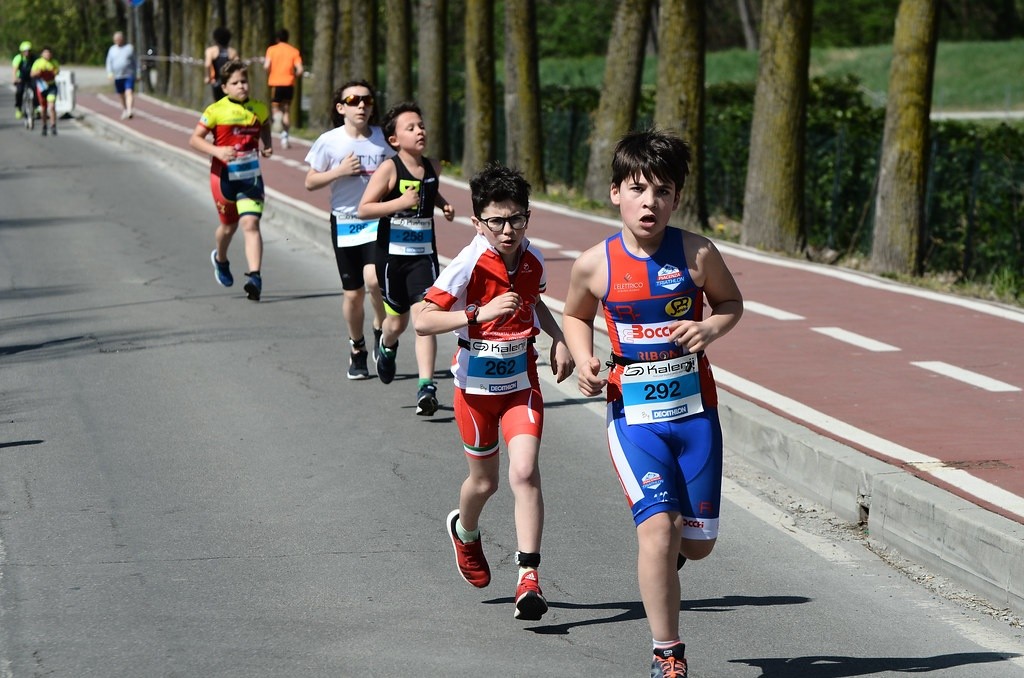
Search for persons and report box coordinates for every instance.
[12,41,60,136]
[562,126,744,678]
[264,28,304,149]
[359,103,455,417]
[204,26,239,102]
[305,80,397,380]
[189,60,274,301]
[414,160,577,620]
[105,31,141,119]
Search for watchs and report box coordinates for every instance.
[463,302,478,326]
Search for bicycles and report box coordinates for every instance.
[20,80,35,130]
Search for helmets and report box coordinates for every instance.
[19,41,32,52]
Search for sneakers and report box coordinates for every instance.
[446,509,491,588]
[513,570,549,622]
[376,333,399,385]
[244,273,262,300]
[372,326,382,363]
[677,552,687,571]
[416,384,439,416]
[210,249,234,288]
[651,641,688,678]
[347,349,369,379]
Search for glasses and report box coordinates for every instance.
[477,210,530,233]
[341,95,376,106]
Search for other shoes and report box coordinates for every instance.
[51,124,56,133]
[121,110,133,119]
[41,125,47,136]
[34,109,41,114]
[15,107,23,119]
[278,131,288,150]
[273,111,283,132]
